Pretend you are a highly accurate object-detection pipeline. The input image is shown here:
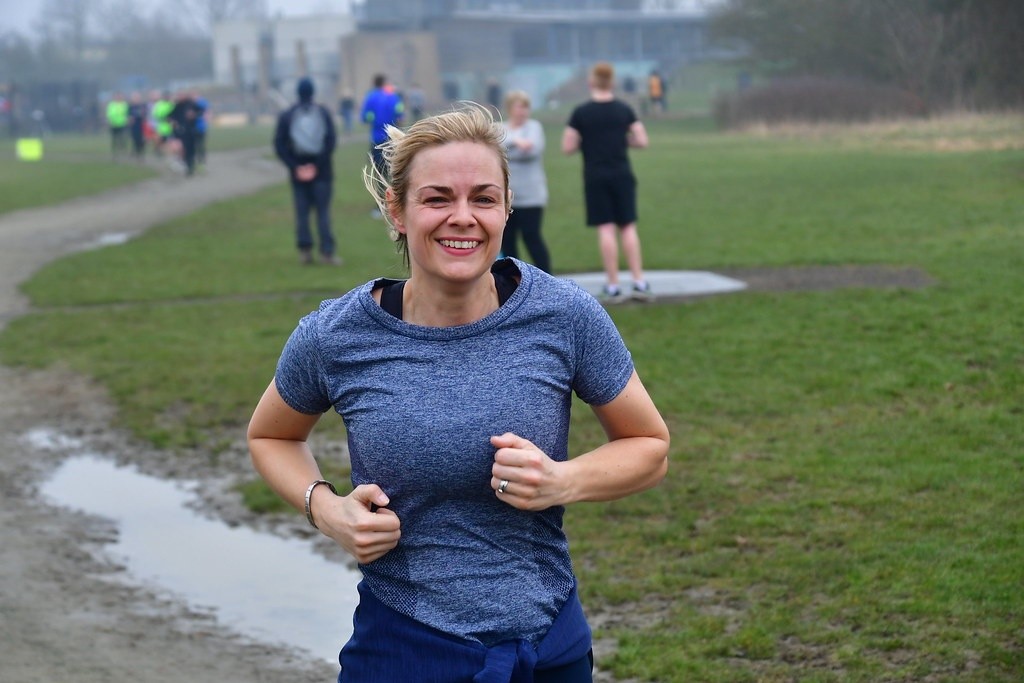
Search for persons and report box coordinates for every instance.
[563,60,658,304]
[272,77,343,267]
[489,88,553,277]
[360,71,408,221]
[0,61,670,177]
[246,98,670,682]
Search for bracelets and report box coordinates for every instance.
[305,479,339,531]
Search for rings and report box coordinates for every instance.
[498,478,510,494]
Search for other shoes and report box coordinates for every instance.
[632,282,657,304]
[320,255,342,265]
[298,248,314,265]
[599,286,626,304]
